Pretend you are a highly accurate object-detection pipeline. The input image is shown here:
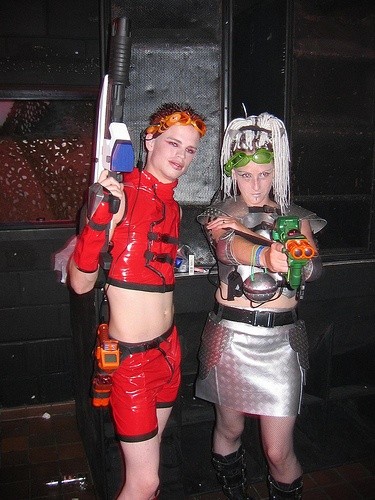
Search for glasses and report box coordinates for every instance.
[161,112,206,138]
[231,151,274,167]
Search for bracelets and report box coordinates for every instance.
[250,243,271,283]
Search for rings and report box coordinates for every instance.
[222,219,225,224]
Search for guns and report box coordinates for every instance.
[93,16,134,230]
[269,215,314,289]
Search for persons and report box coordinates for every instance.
[195,103,330,500]
[67,101,210,500]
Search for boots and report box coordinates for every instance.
[209,442,252,500]
[266,469,304,500]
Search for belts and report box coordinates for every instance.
[213,299,299,328]
